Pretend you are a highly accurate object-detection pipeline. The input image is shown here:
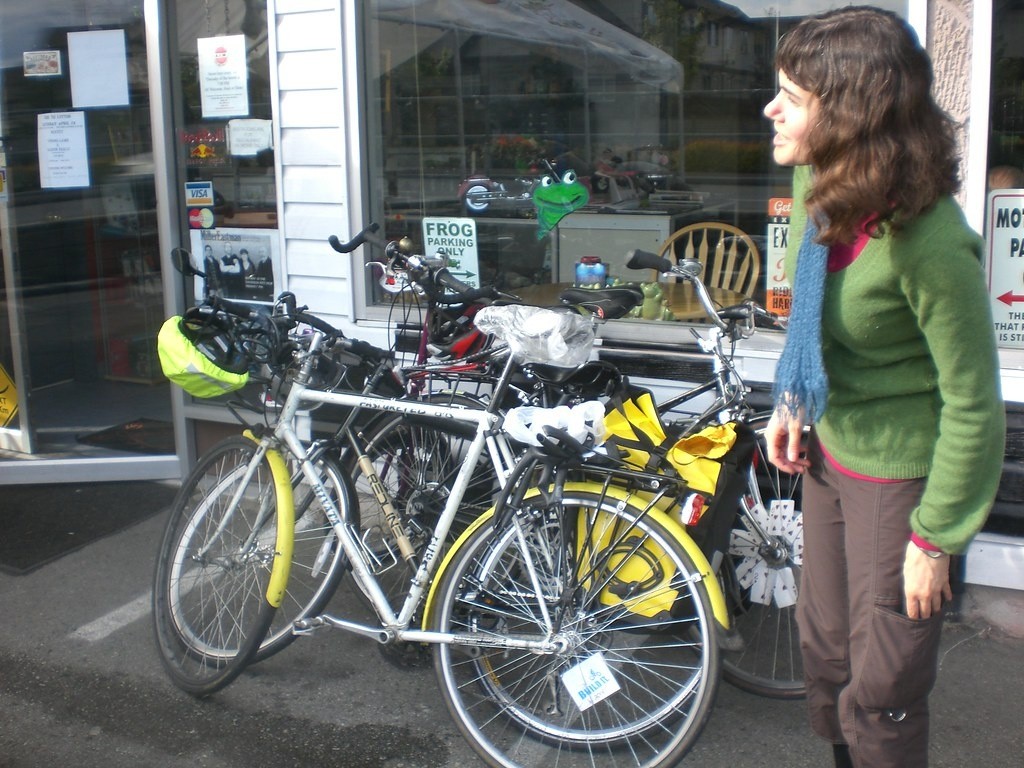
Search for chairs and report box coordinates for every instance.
[648,221,761,299]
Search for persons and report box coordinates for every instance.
[764,5,1007,768]
[203,241,274,300]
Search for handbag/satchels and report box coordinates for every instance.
[572,390,757,633]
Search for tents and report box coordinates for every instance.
[370,0,684,217]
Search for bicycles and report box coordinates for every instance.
[154,224,810,768]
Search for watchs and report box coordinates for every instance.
[920,546,943,559]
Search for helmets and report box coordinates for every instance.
[157,315,249,399]
[263,347,347,410]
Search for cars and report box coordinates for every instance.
[457,139,703,214]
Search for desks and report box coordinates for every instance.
[494,279,749,324]
